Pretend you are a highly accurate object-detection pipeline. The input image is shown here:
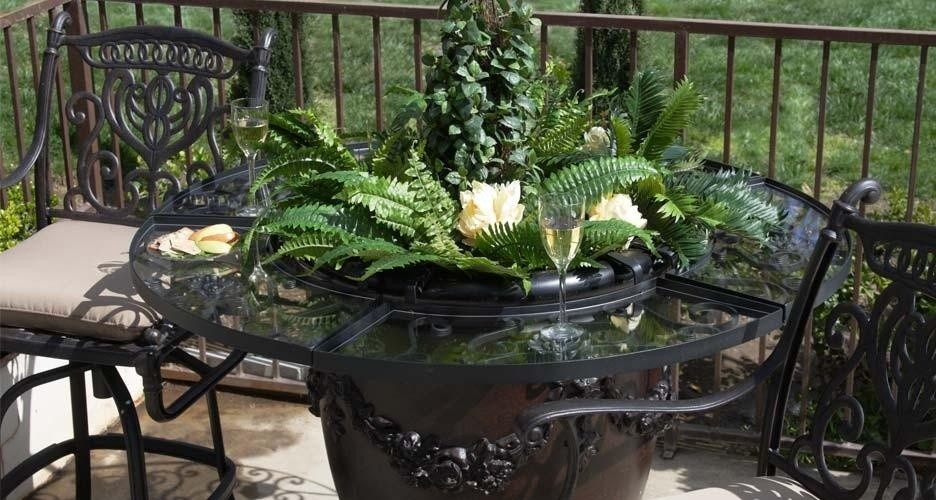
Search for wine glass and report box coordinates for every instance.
[230,98,269,217]
[537,193,587,339]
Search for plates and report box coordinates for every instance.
[147,232,240,261]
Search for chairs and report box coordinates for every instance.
[646,174,935,500]
[1,12,287,500]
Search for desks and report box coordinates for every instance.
[126,129,858,499]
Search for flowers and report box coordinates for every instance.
[247,0,791,293]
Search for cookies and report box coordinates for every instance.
[150,227,202,257]
